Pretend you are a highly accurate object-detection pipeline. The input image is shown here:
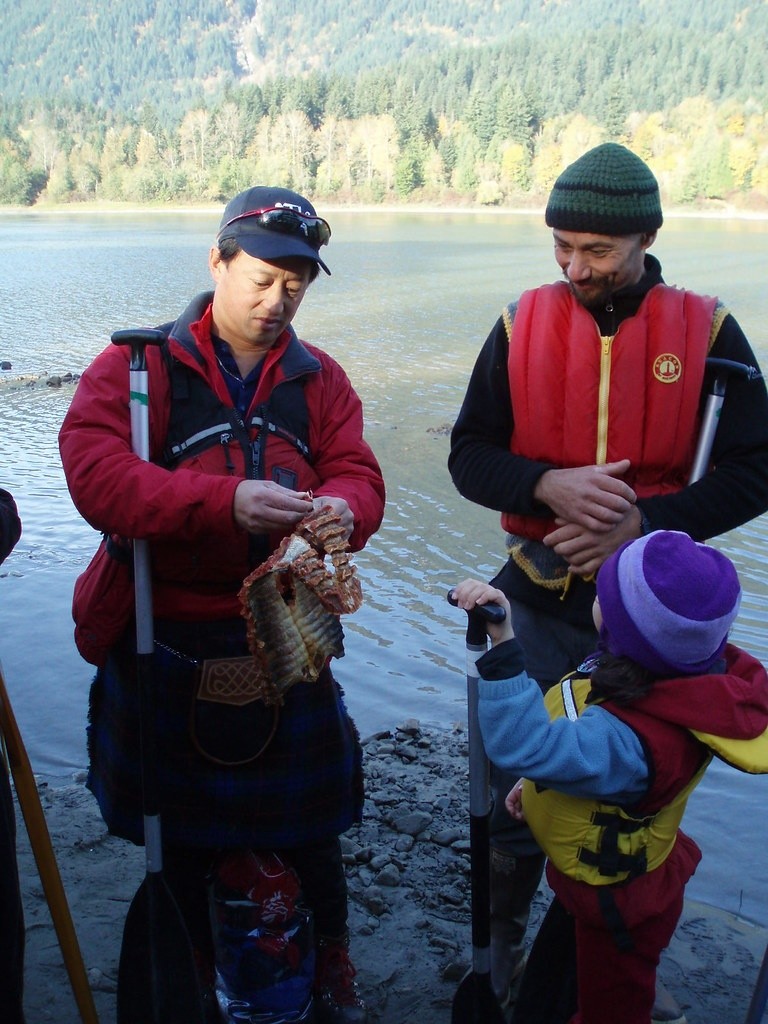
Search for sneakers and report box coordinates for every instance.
[312,930,366,1023]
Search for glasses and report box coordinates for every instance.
[216,208,332,251]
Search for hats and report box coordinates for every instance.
[597,528,742,674]
[219,186,332,279]
[545,142,664,237]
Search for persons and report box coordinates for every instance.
[450,527,768,1024]
[443,139,768,1024]
[54,184,390,1024]
[0,490,27,1024]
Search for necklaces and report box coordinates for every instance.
[214,354,243,383]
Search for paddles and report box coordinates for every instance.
[683,358,759,498]
[115,320,228,1024]
[444,586,507,1024]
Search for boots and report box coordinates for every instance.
[649,978,687,1024]
[452,847,547,1024]
